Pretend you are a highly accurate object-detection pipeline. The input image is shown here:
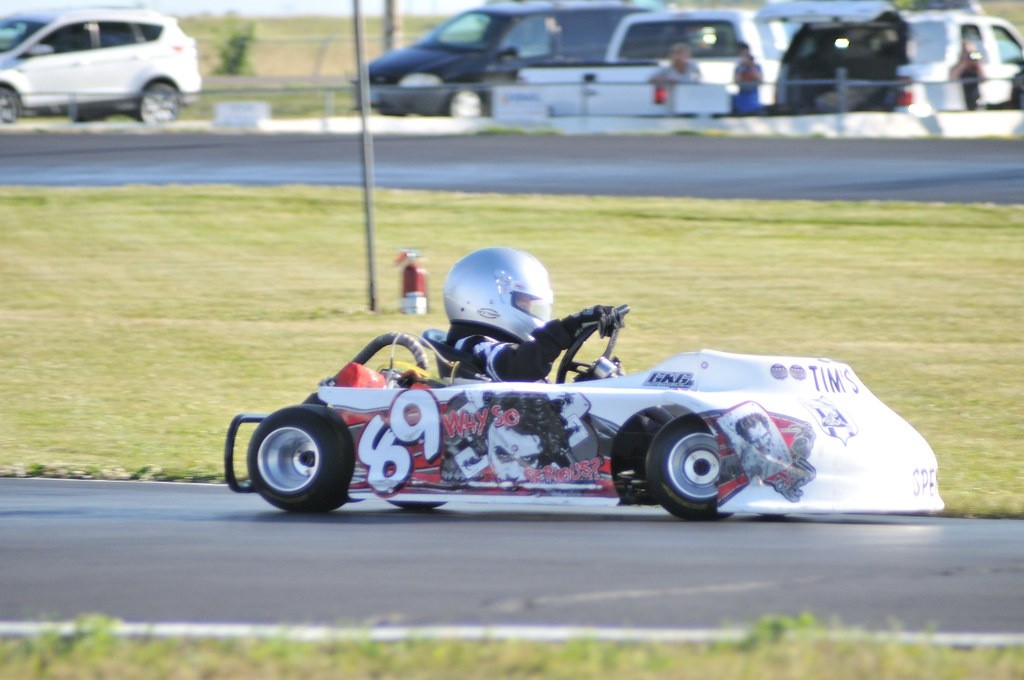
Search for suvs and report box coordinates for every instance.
[0,9,203,125]
[774,7,1024,118]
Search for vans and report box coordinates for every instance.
[353,0,686,118]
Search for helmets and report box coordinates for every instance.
[443,248,553,341]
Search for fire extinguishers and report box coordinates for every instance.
[393,253,431,317]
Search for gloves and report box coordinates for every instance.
[562,304,624,339]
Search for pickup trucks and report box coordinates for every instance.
[491,8,804,116]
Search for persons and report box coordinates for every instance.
[652,42,702,83]
[948,38,987,108]
[733,55,763,94]
[422,247,625,385]
